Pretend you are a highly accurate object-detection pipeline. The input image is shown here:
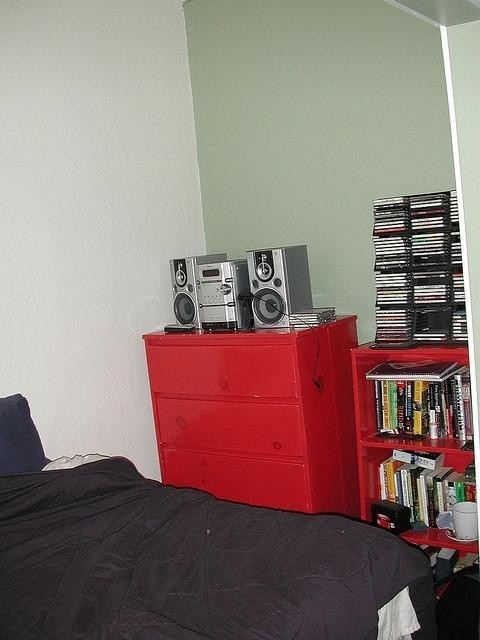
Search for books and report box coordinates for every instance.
[364,360,479,531]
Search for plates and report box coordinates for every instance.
[445,530,478,542]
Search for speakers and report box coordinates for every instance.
[169,252,229,329]
[245,244,314,328]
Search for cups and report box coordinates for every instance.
[451,501,477,540]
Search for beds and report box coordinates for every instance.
[1,393,441,639]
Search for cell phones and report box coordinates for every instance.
[164,323,195,333]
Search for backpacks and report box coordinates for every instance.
[436,562,479,639]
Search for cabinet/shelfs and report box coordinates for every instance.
[141,314,360,519]
[348,336,480,567]
